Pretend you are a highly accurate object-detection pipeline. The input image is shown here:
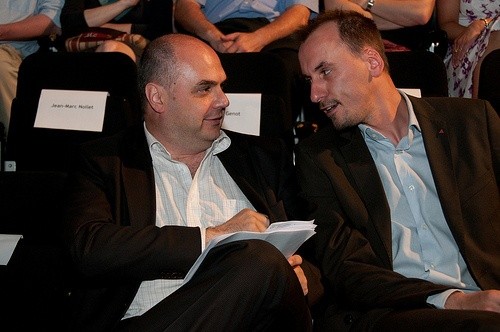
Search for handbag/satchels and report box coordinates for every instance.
[65,27,150,56]
[378,26,449,56]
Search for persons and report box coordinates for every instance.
[49,33,325,332]
[294,7,500,332]
[436,0,500,116]
[324,0,436,97]
[174,0,320,95]
[4,0,174,174]
[0,0,65,148]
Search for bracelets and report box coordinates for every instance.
[365,0,375,11]
[480,18,488,29]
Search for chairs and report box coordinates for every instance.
[0,51,500,332]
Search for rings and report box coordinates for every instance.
[453,49,458,53]
[458,48,463,51]
